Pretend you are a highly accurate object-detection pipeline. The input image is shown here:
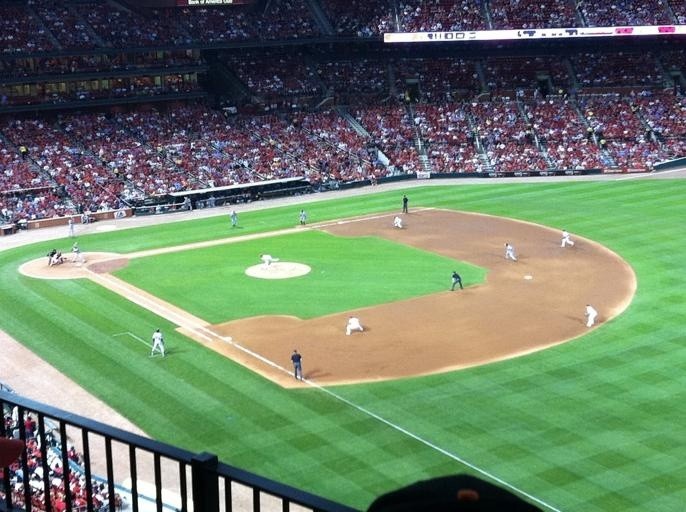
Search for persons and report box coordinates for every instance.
[150,328,165,357]
[584,304,598,328]
[345,316,363,335]
[449,271,463,292]
[0,405,130,512]
[290,349,303,380]
